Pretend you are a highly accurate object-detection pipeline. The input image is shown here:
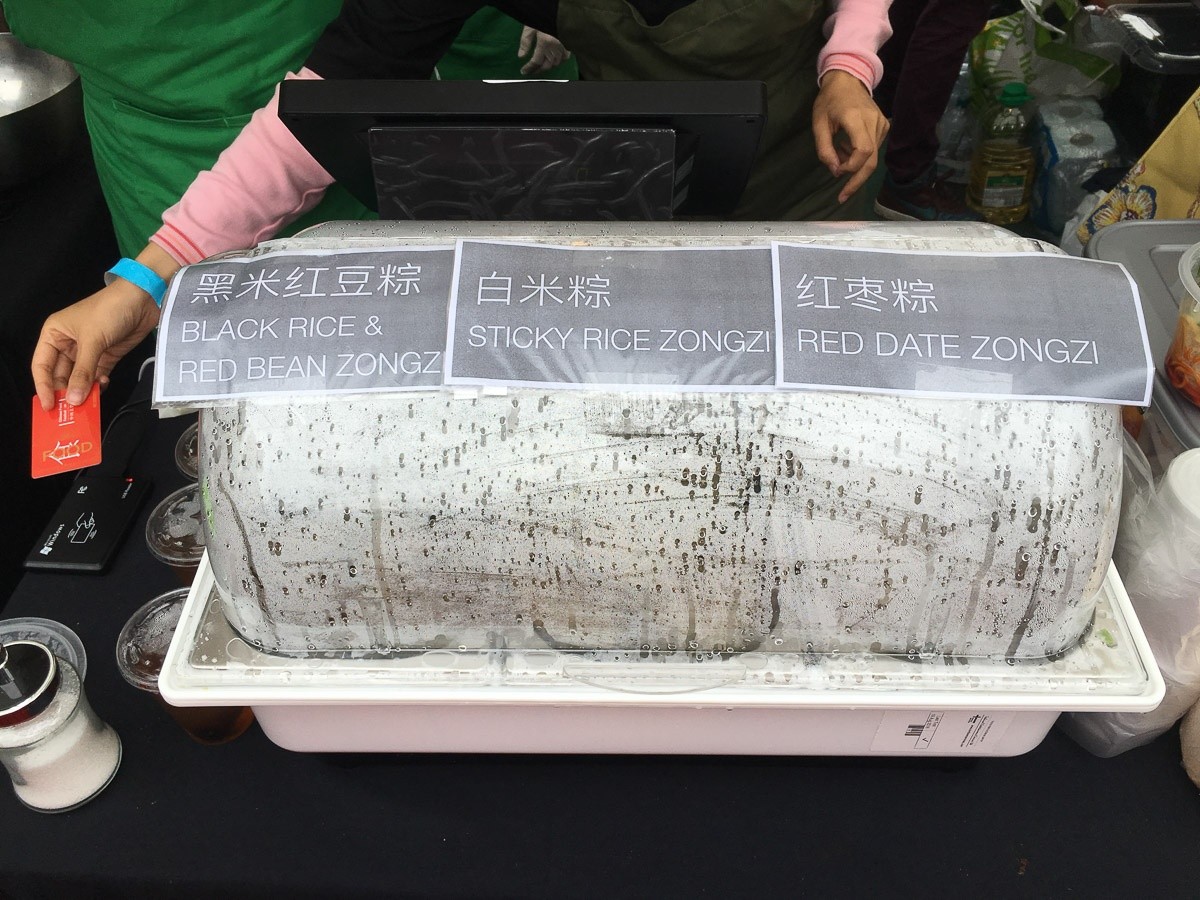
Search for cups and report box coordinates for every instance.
[172,417,202,484]
[116,582,258,746]
[146,482,209,585]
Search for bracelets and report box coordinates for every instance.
[102,258,168,308]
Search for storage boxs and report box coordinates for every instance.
[1088,218,1200,482]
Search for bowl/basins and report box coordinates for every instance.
[1164,240,1200,408]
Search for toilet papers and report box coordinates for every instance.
[1034,97,1103,230]
[1047,121,1117,234]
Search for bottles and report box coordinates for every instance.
[0,640,123,814]
[963,81,1039,225]
[1058,447,1200,758]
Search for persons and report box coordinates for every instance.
[31,0,892,413]
[0,0,580,339]
